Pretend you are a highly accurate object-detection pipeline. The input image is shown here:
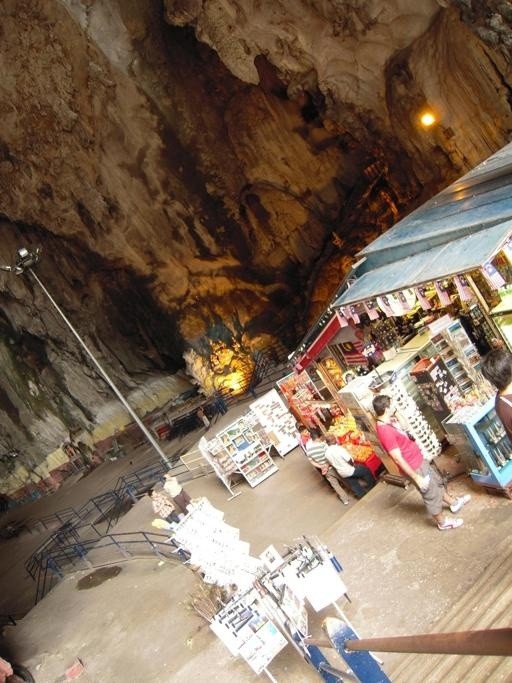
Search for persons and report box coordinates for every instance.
[372,394,472,531]
[149,489,178,524]
[481,349,512,448]
[195,408,211,426]
[162,474,194,511]
[291,420,378,506]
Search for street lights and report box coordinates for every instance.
[1,242,176,472]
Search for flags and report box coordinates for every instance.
[330,265,505,328]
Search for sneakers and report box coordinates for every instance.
[450,494,471,513]
[438,516,463,530]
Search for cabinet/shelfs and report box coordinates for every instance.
[441,393,512,500]
[198,411,278,501]
